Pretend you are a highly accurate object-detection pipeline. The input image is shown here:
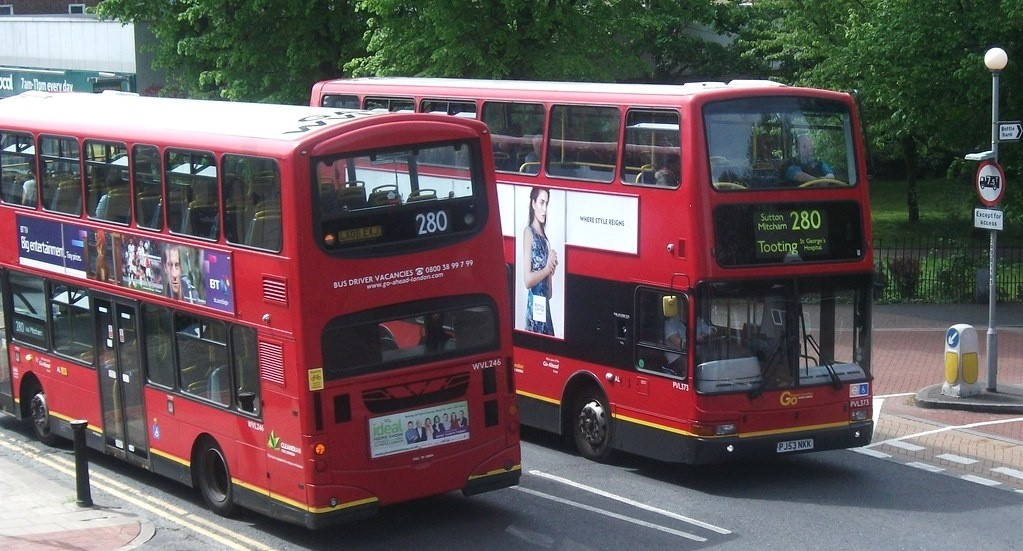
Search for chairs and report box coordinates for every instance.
[454,146,634,183]
[53,306,454,405]
[0,157,438,248]
[635,121,783,189]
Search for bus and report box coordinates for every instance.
[310,77,876,466]
[0,90,522,529]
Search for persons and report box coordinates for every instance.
[405,419,427,444]
[96,174,124,218]
[664,297,715,376]
[523,188,557,336]
[424,410,467,439]
[161,243,183,301]
[125,237,153,287]
[492,129,516,170]
[524,129,555,173]
[21,157,58,208]
[782,135,835,185]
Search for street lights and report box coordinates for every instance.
[984,47,1007,391]
